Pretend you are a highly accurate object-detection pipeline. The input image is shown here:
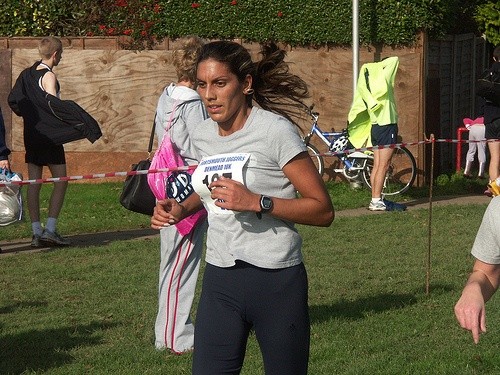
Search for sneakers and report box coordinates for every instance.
[484,178,500,198]
[368,197,408,211]
[30,233,57,248]
[39,226,71,247]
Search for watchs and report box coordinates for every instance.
[256,195,272,220]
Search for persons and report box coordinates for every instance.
[463,117,487,178]
[0,108,11,169]
[154,35,210,355]
[152,40,335,375]
[366,56,399,210]
[454,195,500,344]
[482,46,500,196]
[20,35,69,248]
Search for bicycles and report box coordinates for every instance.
[299,102,417,197]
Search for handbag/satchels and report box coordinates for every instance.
[120,157,156,216]
[146,133,207,237]
